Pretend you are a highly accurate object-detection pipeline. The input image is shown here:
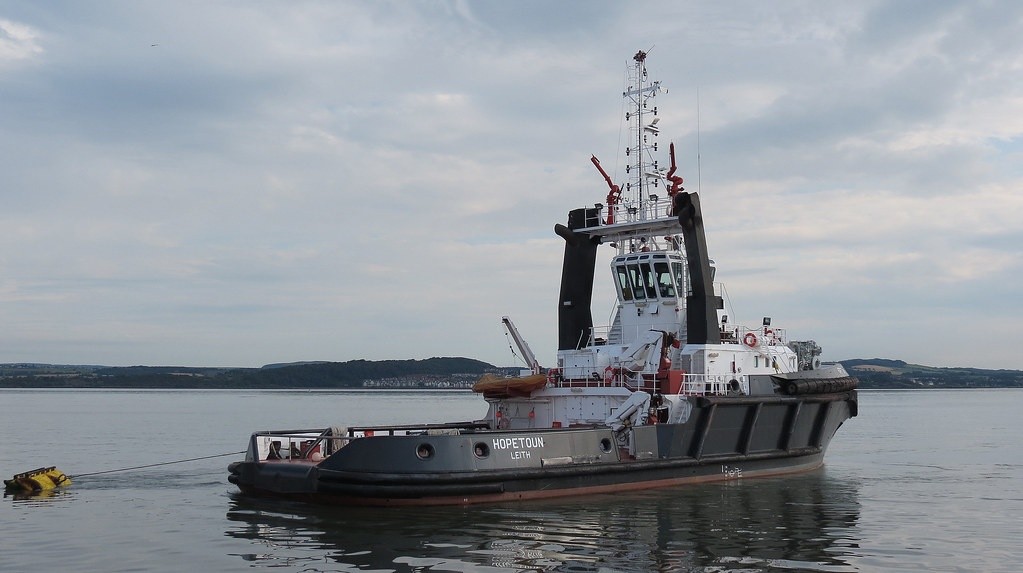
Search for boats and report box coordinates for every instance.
[227,45,859,506]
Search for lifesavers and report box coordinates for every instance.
[547,368,558,383]
[766,330,776,339]
[602,366,615,382]
[743,332,757,347]
